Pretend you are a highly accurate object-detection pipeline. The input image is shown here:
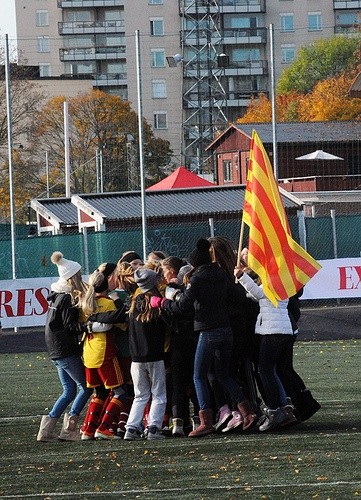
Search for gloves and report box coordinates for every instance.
[150,297,167,309]
[91,322,113,332]
[165,287,180,301]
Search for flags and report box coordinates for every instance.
[242,131,323,308]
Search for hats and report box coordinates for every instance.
[134,268,160,293]
[51,252,81,281]
[187,238,212,268]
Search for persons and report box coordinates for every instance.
[35,236,321,442]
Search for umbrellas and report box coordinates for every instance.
[146,167,217,189]
[294,150,345,175]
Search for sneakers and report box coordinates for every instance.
[173,397,294,439]
[145,426,167,440]
[123,429,143,439]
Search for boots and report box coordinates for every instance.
[59,412,81,441]
[79,390,128,440]
[295,390,320,421]
[37,415,59,442]
